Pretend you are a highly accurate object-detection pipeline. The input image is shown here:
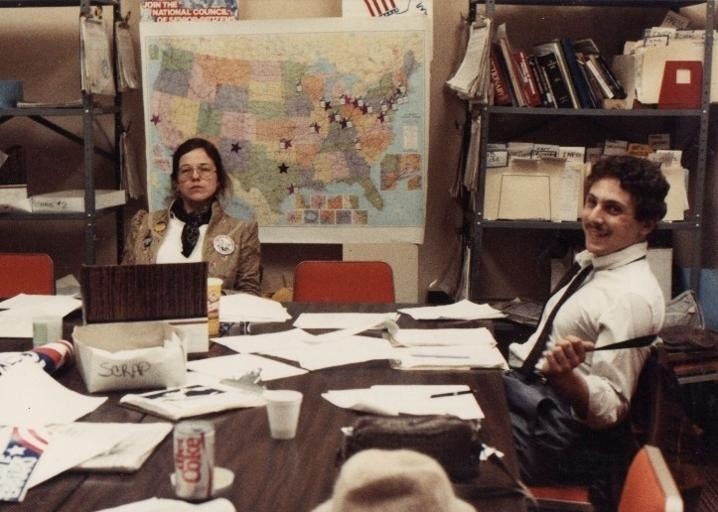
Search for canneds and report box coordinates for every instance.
[173,420,216,500]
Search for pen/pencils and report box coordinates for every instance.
[430,388,478,398]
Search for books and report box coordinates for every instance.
[488,33,625,109]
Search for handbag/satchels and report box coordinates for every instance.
[337,414,479,482]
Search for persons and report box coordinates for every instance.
[122,137,263,320]
[501,154,666,489]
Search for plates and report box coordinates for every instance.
[172,466,236,499]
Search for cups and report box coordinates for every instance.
[207,278,225,335]
[263,389,303,440]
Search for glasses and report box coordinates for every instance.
[177,164,218,180]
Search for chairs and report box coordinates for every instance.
[616,443,686,511]
[0,251,55,297]
[291,260,396,304]
[517,341,669,512]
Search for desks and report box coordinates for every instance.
[0,320,235,512]
[54,299,532,512]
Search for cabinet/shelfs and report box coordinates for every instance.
[455,0,716,331]
[0,1,126,268]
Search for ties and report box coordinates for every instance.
[522,261,594,386]
